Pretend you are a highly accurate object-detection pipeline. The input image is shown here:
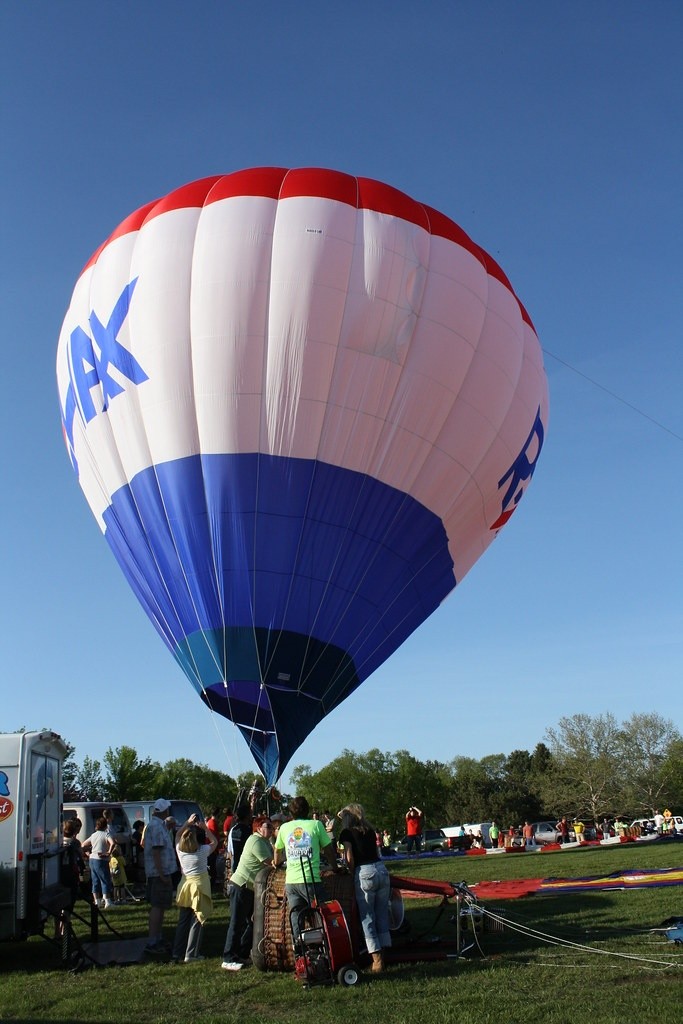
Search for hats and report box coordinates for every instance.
[153,797,172,813]
[561,816,567,819]
[572,817,579,821]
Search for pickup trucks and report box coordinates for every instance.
[397,830,463,853]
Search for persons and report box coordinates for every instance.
[600,817,623,840]
[647,810,677,835]
[375,828,391,855]
[509,819,536,846]
[313,810,337,848]
[222,804,285,970]
[82,809,127,909]
[406,806,423,855]
[336,802,392,972]
[174,812,219,962]
[205,806,233,883]
[572,817,586,842]
[140,798,178,954]
[489,821,503,848]
[459,827,483,847]
[274,796,348,964]
[54,815,82,939]
[556,816,570,843]
[132,820,146,889]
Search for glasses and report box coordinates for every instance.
[261,824,274,829]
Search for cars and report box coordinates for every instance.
[530,821,592,846]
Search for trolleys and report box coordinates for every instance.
[289,854,364,990]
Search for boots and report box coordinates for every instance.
[358,951,384,975]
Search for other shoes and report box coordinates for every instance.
[220,961,244,971]
[184,955,206,963]
[145,939,172,954]
[105,903,118,909]
[172,955,184,961]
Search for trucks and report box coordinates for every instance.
[0,727,71,943]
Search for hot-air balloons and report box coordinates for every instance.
[56,165,550,973]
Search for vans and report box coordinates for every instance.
[120,800,206,843]
[628,816,683,837]
[62,802,136,868]
